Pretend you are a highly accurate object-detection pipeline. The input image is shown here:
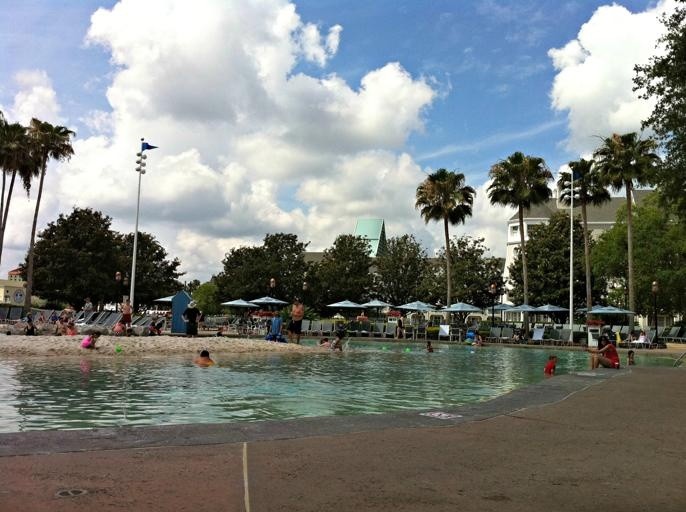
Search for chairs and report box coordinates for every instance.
[485,327,513,344]
[404,324,427,340]
[297,319,396,339]
[42,309,166,337]
[529,327,570,346]
[0,307,22,325]
[437,325,460,344]
[621,326,686,349]
[227,321,267,335]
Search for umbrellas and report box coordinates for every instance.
[247,295,289,320]
[577,305,607,312]
[219,297,260,317]
[154,295,176,302]
[587,305,636,329]
[326,298,570,320]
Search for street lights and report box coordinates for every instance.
[114,271,121,314]
[302,280,307,305]
[563,181,581,340]
[652,280,658,343]
[489,284,496,326]
[129,139,146,313]
[269,278,275,299]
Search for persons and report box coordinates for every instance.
[394,313,406,339]
[264,298,304,345]
[426,341,433,352]
[544,354,557,378]
[216,326,223,337]
[466,317,482,347]
[7,297,101,351]
[79,359,93,386]
[181,301,201,338]
[113,299,172,337]
[319,322,347,353]
[582,330,650,369]
[193,350,215,366]
